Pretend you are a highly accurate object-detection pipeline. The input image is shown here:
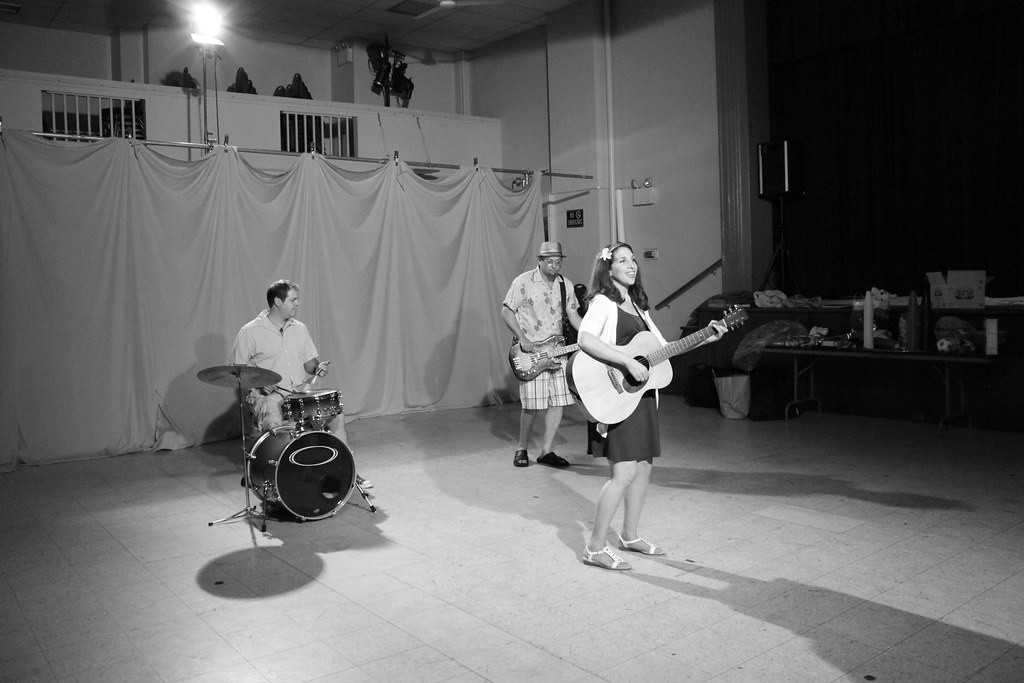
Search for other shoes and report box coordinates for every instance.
[351,474,373,489]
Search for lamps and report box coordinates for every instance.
[336,39,353,65]
[367,33,414,108]
[630,178,656,207]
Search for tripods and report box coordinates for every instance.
[209,372,263,527]
[759,196,802,295]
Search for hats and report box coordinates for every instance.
[537,241,567,258]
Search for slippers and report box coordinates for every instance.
[537,452,569,468]
[514,450,529,467]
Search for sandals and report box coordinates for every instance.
[617,534,664,556]
[582,544,632,570]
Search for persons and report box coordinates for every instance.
[230,280,374,489]
[577,243,728,570]
[502,242,583,469]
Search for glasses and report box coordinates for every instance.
[541,257,561,267]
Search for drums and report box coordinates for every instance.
[247,426,357,521]
[283,389,344,422]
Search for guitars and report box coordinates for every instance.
[565,303,750,425]
[508,334,580,383]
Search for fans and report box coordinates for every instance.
[413,0,504,19]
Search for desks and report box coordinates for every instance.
[747,343,992,439]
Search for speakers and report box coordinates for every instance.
[758,138,803,196]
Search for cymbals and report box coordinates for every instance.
[196,365,283,390]
